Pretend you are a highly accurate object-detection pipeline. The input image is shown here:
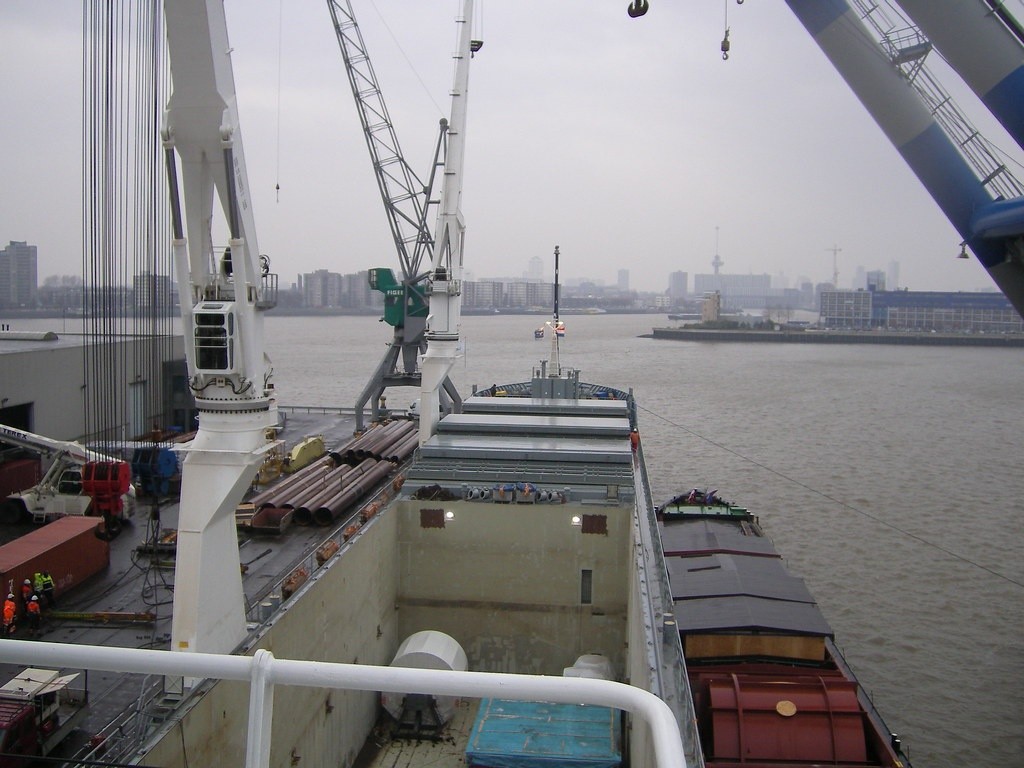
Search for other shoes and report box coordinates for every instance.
[29,629,34,635]
[37,630,43,637]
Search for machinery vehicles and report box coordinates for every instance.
[0,423,139,539]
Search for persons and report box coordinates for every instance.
[23,570,55,638]
[4,594,16,638]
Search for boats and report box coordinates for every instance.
[534,328,545,338]
[557,326,566,337]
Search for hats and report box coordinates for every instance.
[8,594,14,598]
[24,579,30,584]
[31,595,38,600]
